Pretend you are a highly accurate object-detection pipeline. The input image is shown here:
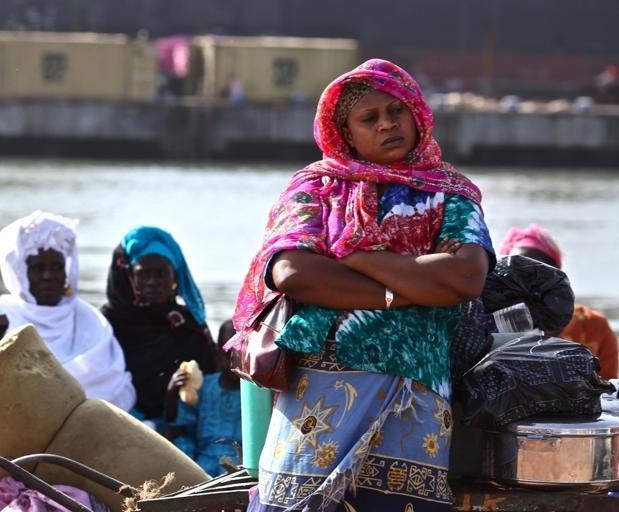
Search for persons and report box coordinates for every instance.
[0,208,136,416]
[100,223,217,444]
[498,223,619,380]
[234,56,499,512]
[171,316,244,480]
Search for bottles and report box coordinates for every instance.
[482,303,535,335]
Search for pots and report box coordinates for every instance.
[481,409,618,495]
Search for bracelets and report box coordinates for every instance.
[384,289,394,308]
